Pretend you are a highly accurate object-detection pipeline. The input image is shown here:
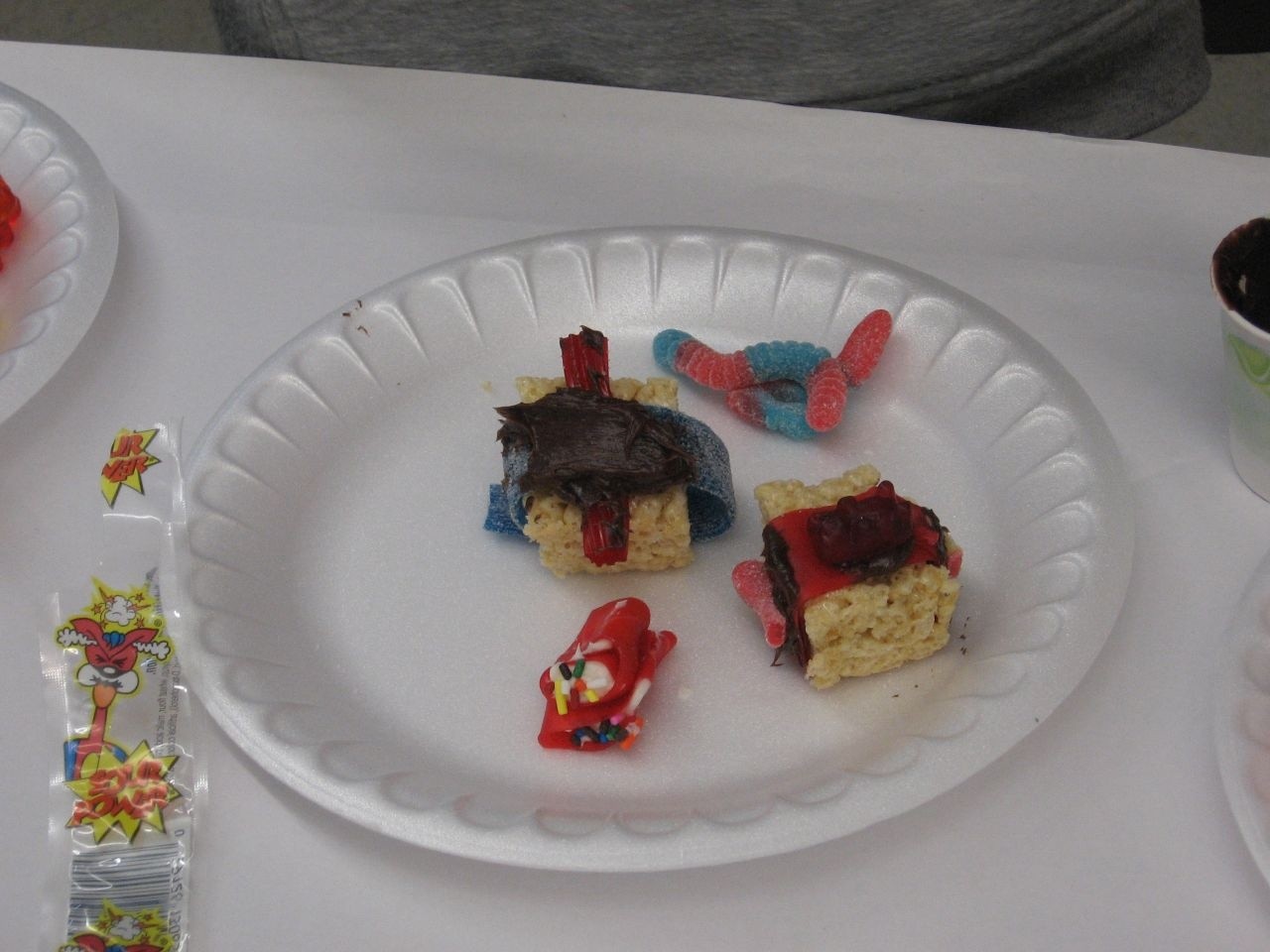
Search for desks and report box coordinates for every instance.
[1,44,1269,952]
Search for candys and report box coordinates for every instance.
[649,310,896,440]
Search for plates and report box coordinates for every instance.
[157,223,1135,877]
[1214,548,1270,890]
[0,82,121,424]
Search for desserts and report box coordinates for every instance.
[481,327,965,694]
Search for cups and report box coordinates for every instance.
[1210,216,1269,502]
